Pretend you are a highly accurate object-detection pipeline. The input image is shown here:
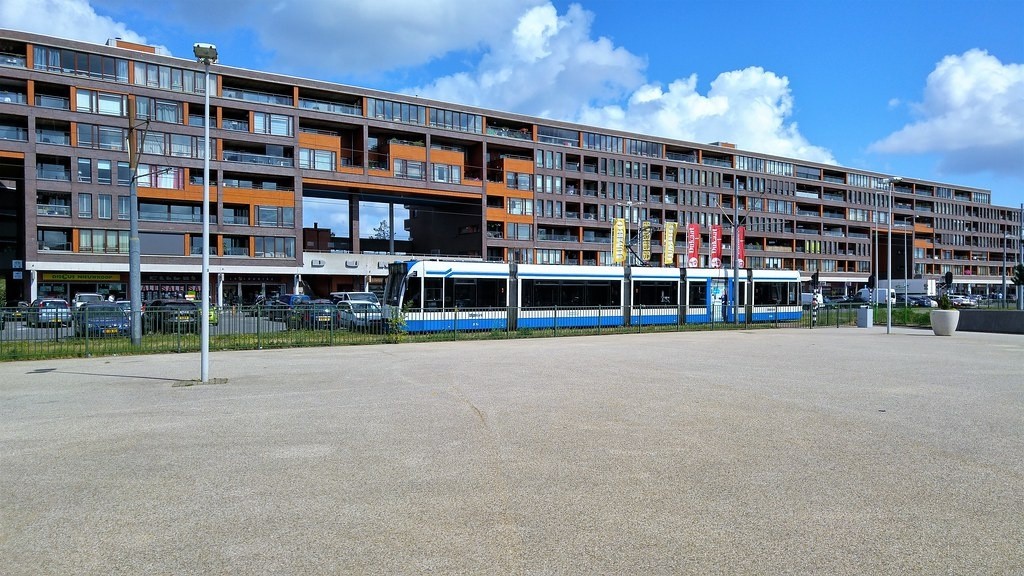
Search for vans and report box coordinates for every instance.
[72,293,104,318]
[250,293,310,323]
[329,291,381,312]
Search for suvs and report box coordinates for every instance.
[28,298,72,328]
[141,299,201,334]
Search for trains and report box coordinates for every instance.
[381,258,806,334]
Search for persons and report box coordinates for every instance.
[108,294,115,303]
[823,295,831,311]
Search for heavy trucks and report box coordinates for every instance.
[855,279,937,303]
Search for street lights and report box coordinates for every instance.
[1003,230,1012,308]
[880,177,903,335]
[193,41,219,376]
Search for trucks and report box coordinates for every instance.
[853,288,896,308]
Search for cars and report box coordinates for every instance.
[830,296,848,302]
[295,299,339,330]
[116,301,145,322]
[948,291,1018,307]
[191,300,219,326]
[73,300,131,339]
[896,294,941,308]
[336,300,384,333]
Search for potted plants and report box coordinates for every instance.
[929,293,960,336]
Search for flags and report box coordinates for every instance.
[611,217,626,262]
[639,220,652,262]
[731,226,745,269]
[688,224,699,268]
[709,225,722,269]
[664,222,678,264]
